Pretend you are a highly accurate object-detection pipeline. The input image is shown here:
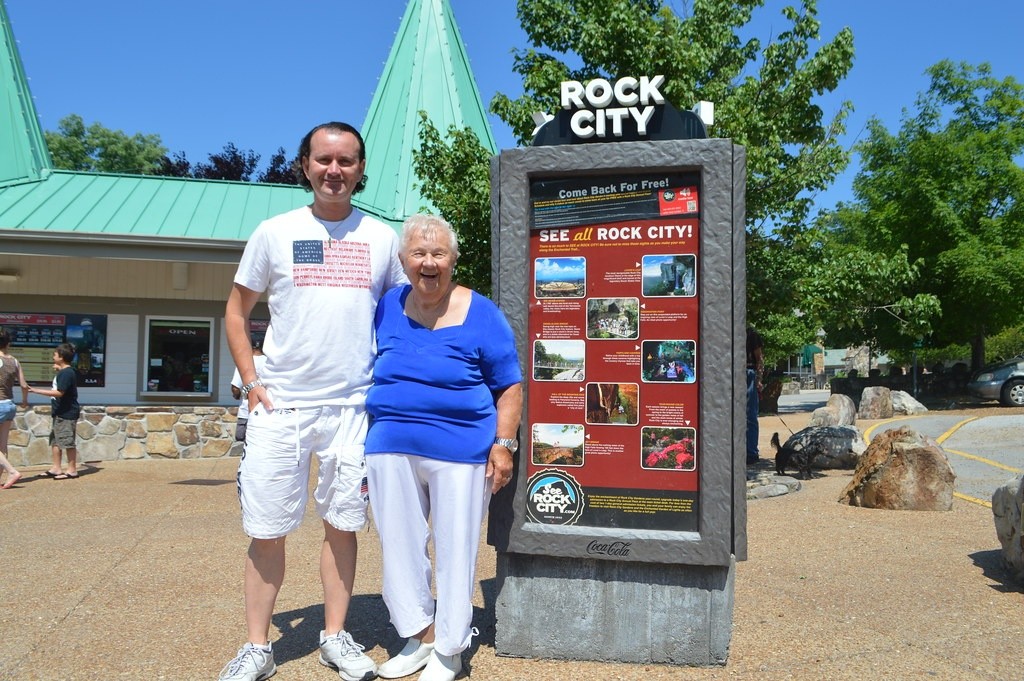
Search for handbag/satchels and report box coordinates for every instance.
[235,418,248,441]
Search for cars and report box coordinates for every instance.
[967,355,1024,407]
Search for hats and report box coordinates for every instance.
[251,334,264,349]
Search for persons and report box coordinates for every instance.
[215,123,413,681]
[0,329,29,489]
[746,327,763,464]
[364,214,522,680]
[27,343,80,480]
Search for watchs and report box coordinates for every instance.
[242,381,262,399]
[495,438,518,453]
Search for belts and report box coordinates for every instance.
[0,398,14,403]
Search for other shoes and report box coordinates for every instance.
[747,455,759,464]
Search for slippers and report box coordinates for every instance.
[0,473,22,488]
[38,471,66,478]
[54,473,79,480]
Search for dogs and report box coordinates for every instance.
[770,433,829,480]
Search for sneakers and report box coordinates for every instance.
[319,630,378,681]
[417,648,462,681]
[377,637,434,678]
[218,641,277,681]
